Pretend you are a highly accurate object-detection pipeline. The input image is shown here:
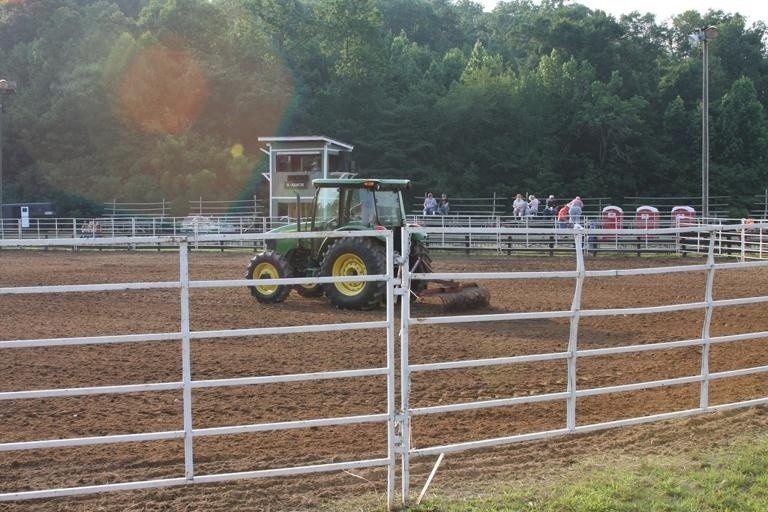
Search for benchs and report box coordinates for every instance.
[621,191,731,229]
[180,195,265,232]
[748,189,768,234]
[409,192,508,227]
[99,198,173,233]
[511,191,613,227]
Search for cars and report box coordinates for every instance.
[183,215,237,232]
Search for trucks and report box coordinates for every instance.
[2,202,57,230]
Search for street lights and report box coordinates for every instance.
[686,22,718,224]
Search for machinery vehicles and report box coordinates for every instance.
[245,176,431,312]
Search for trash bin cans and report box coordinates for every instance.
[602,206,624,242]
[635,206,660,242]
[670,206,696,243]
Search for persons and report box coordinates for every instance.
[566,196,584,224]
[512,193,528,220]
[437,193,450,215]
[422,192,438,215]
[558,205,569,229]
[544,194,559,215]
[525,194,541,219]
